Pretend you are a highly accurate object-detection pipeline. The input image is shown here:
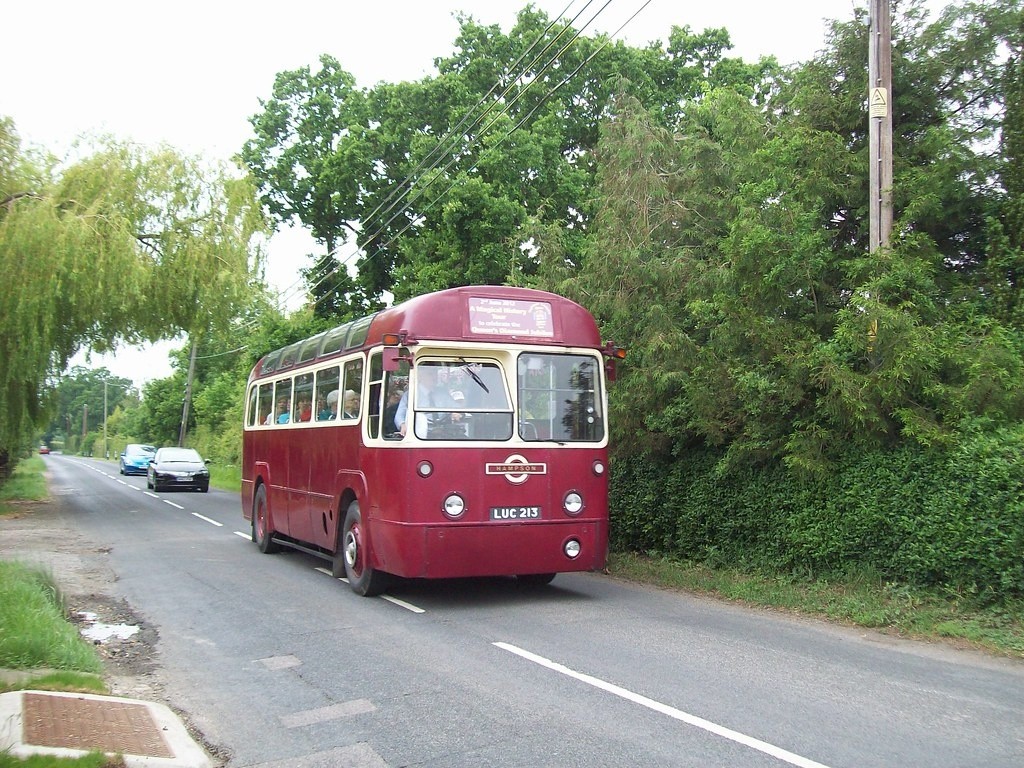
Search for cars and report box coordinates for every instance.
[118,444,159,475]
[146,447,212,492]
[40,445,49,454]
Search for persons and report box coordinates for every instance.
[260,389,358,425]
[395,362,465,436]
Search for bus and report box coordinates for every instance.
[239,287,624,596]
